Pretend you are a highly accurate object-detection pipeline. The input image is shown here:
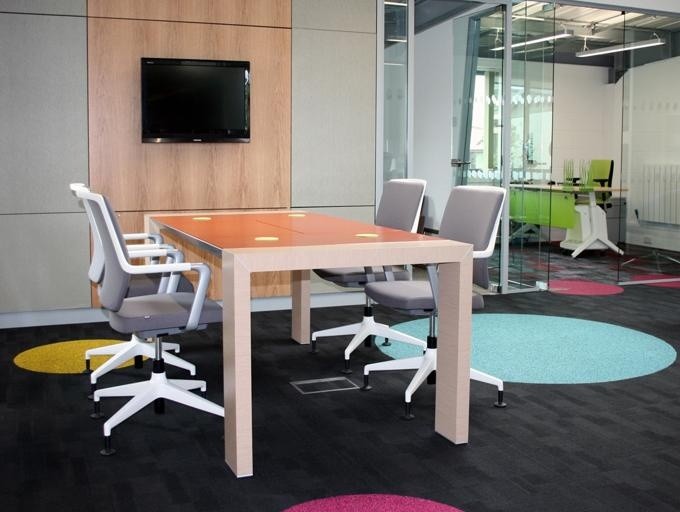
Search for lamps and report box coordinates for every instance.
[489,23,575,52]
[575,30,668,60]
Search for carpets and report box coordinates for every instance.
[373,311,677,387]
[541,278,625,297]
[632,274,680,289]
[12,339,151,377]
[282,495,472,512]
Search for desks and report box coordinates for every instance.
[499,186,630,259]
[141,205,475,481]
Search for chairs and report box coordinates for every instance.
[308,178,430,357]
[568,160,616,210]
[82,188,225,456]
[360,183,511,420]
[71,182,202,397]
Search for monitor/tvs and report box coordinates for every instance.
[141,57,250,143]
